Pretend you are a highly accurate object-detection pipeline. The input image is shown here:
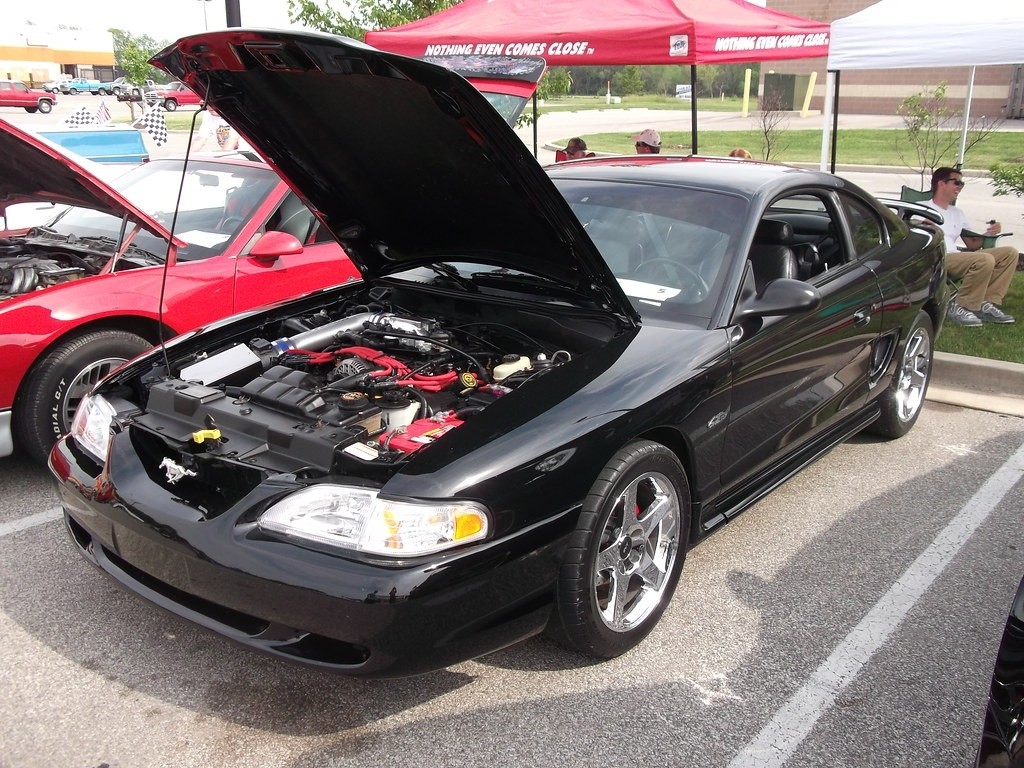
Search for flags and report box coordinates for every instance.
[138,105,168,147]
[62,107,94,129]
[95,102,111,125]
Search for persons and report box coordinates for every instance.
[911,167,1020,327]
[189,102,241,156]
[560,137,588,160]
[728,147,753,161]
[629,128,662,157]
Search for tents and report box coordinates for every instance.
[364,0,841,174]
[822,0,1024,176]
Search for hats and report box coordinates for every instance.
[561,137,587,154]
[632,129,662,147]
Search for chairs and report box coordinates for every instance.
[898,185,1013,302]
[748,219,799,293]
[227,183,320,246]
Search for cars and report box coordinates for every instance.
[145,80,208,111]
[49,28,950,682]
[0,81,56,114]
[0,54,549,477]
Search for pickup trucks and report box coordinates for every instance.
[111,76,154,98]
[59,78,113,96]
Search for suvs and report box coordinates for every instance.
[42,78,73,94]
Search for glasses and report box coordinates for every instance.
[946,178,964,186]
[635,142,645,147]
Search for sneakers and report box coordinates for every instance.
[974,301,1015,323]
[947,305,983,326]
